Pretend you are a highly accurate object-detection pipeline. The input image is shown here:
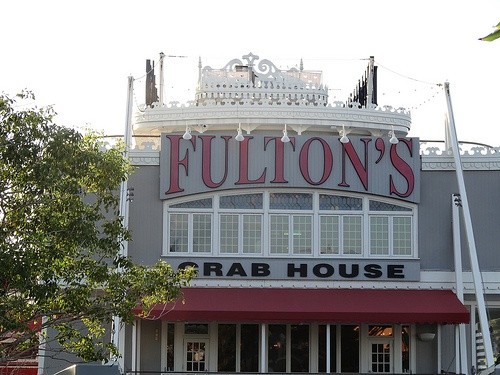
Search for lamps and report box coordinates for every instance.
[281,124,290,144]
[234,123,244,142]
[340,126,349,144]
[182,123,192,140]
[388,127,399,144]
